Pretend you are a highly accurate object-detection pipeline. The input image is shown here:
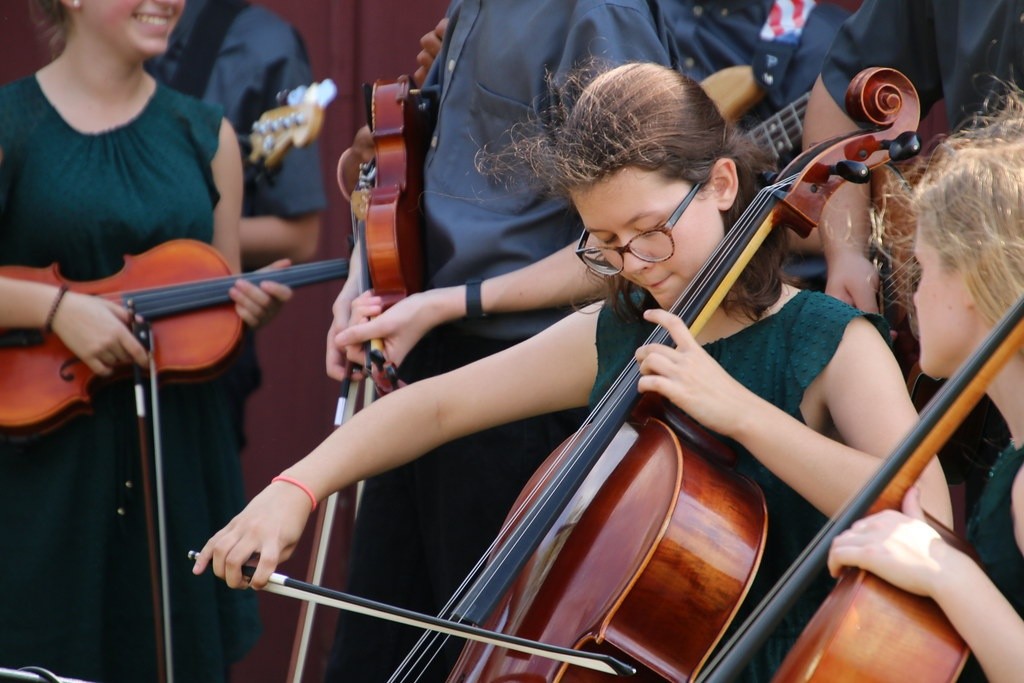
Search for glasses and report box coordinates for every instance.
[575,182,702,276]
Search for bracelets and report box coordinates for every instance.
[465,278,489,319]
[45,283,71,335]
[270,476,317,513]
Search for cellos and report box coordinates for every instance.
[438,66,923,683]
[690,290,1024,683]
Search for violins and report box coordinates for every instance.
[0,237,353,452]
[359,72,418,390]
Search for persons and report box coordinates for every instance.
[325,1,1024,683]
[1,2,324,683]
[191,57,955,683]
[827,79,1024,682]
